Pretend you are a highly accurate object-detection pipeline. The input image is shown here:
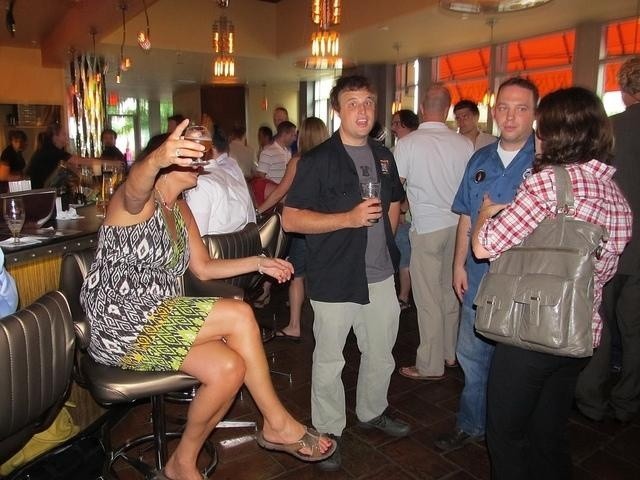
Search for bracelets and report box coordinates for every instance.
[256,254,263,275]
[478,208,490,218]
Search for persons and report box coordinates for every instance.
[436,78,543,451]
[451,100,500,150]
[389,86,474,380]
[256,121,297,185]
[472,87,633,480]
[575,54,640,424]
[0,245,20,323]
[0,130,29,189]
[96,129,126,161]
[80,118,338,480]
[388,110,420,315]
[254,116,330,340]
[273,107,300,157]
[218,121,257,180]
[167,115,186,136]
[256,125,274,171]
[26,123,125,193]
[283,76,411,472]
[183,125,256,236]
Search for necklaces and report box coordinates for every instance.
[153,183,176,210]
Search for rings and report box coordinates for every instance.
[176,149,182,156]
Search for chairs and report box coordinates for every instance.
[255,206,298,382]
[164,223,295,425]
[60,254,218,480]
[0,289,72,480]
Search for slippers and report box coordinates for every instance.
[257,425,337,462]
[272,327,301,341]
[399,365,447,381]
[156,466,209,480]
[397,296,411,310]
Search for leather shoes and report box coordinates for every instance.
[435,429,485,450]
[315,432,342,471]
[366,413,411,437]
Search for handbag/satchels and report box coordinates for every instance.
[473,162,608,358]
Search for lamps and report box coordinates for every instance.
[307,1,343,71]
[137,1,151,51]
[260,82,268,112]
[211,0,236,80]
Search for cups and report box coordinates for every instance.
[359,177,383,224]
[184,125,214,168]
[2,196,27,248]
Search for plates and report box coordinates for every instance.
[1,235,46,250]
